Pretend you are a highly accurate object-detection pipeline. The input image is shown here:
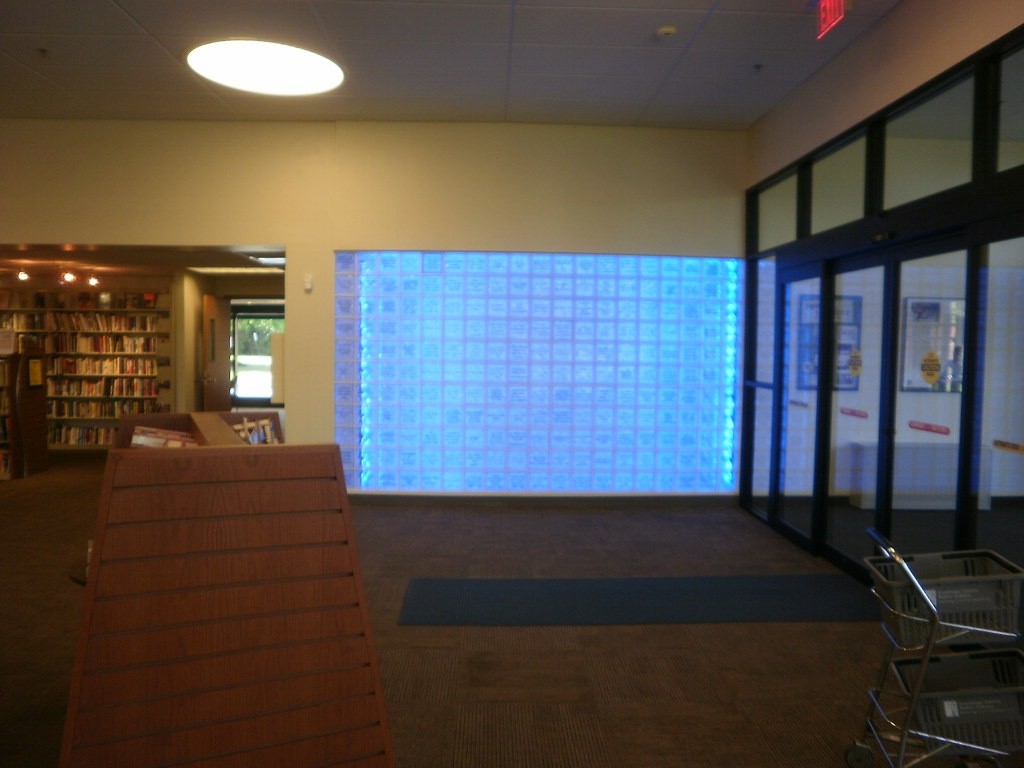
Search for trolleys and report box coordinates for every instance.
[861,524,1024,768]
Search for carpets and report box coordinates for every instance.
[396,574,886,626]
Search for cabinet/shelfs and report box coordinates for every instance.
[0,307,171,454]
[0,335,49,481]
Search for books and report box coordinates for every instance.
[0,285,174,481]
